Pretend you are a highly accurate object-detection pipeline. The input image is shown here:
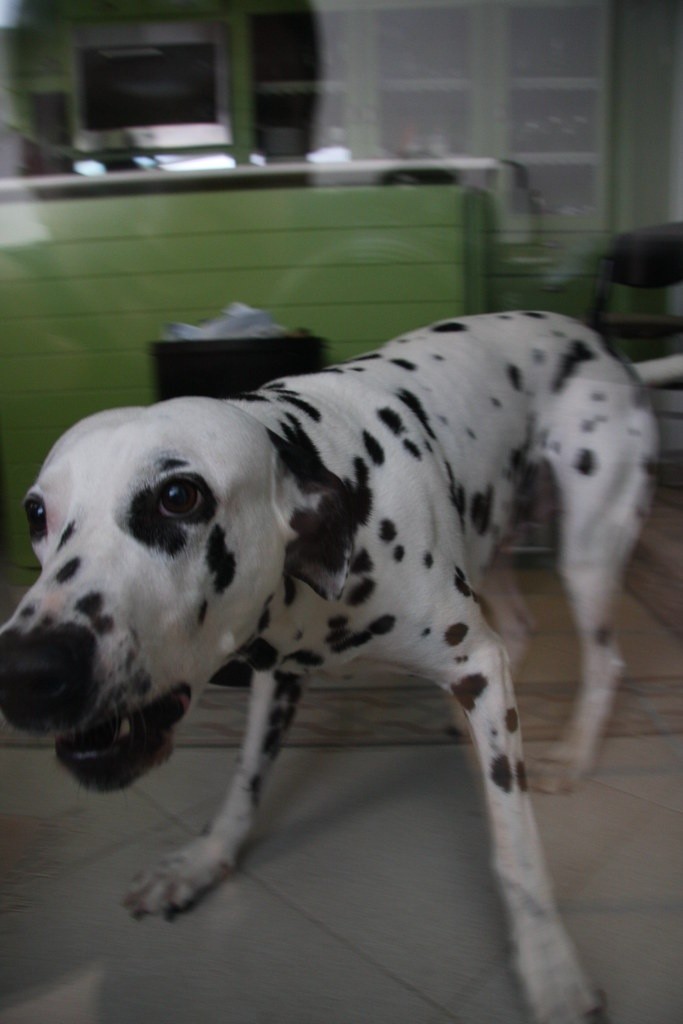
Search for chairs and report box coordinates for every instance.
[513,221,683,528]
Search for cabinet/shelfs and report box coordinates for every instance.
[481,213,666,364]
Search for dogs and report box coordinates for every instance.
[1,307,683,1023]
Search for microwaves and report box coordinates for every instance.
[68,18,235,152]
